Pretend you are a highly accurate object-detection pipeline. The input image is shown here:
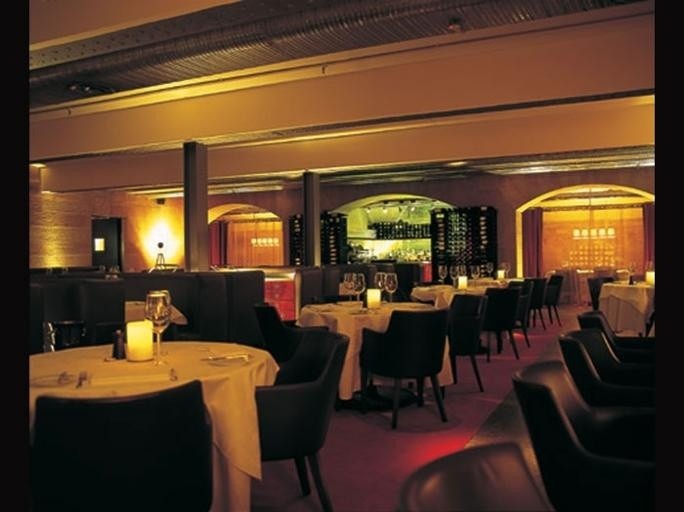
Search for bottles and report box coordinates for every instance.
[110,330,126,360]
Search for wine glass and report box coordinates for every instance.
[437,262,513,289]
[345,271,398,311]
[146,288,173,368]
